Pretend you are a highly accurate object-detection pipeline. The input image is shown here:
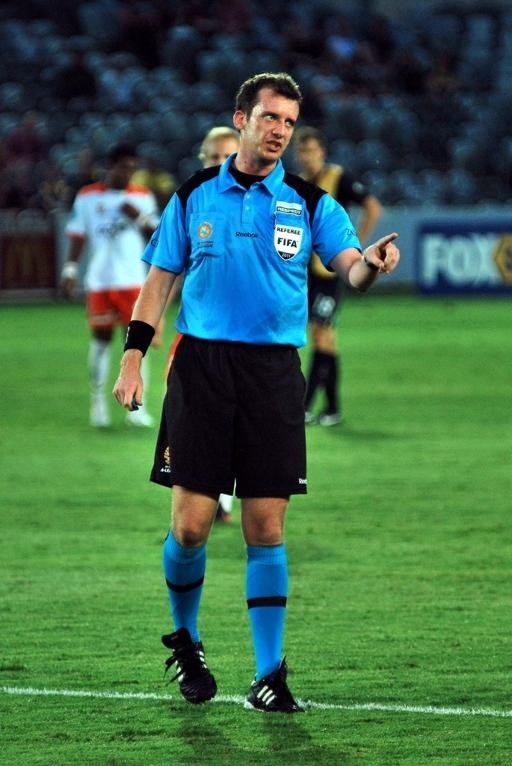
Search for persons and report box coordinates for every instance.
[112,73,401,714]
[57,147,110,204]
[295,129,382,429]
[61,145,162,427]
[136,127,241,524]
[128,156,176,192]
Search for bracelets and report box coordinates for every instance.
[62,262,79,278]
[124,320,156,358]
[134,213,146,229]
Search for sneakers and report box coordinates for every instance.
[124,398,155,431]
[160,625,218,706]
[90,397,114,429]
[245,654,307,714]
